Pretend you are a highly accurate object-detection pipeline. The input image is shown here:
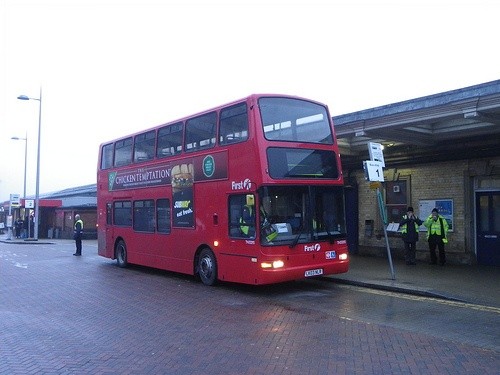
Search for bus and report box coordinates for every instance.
[97,93,351,287]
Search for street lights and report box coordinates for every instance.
[16,85,42,241]
[10,129,27,198]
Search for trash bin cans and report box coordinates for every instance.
[48,226,62,239]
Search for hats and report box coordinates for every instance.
[407,207,413,212]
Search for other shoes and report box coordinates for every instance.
[73,252,82,256]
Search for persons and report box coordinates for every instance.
[73,214,84,256]
[14,214,35,239]
[424,208,449,265]
[399,207,422,265]
[240,199,268,239]
